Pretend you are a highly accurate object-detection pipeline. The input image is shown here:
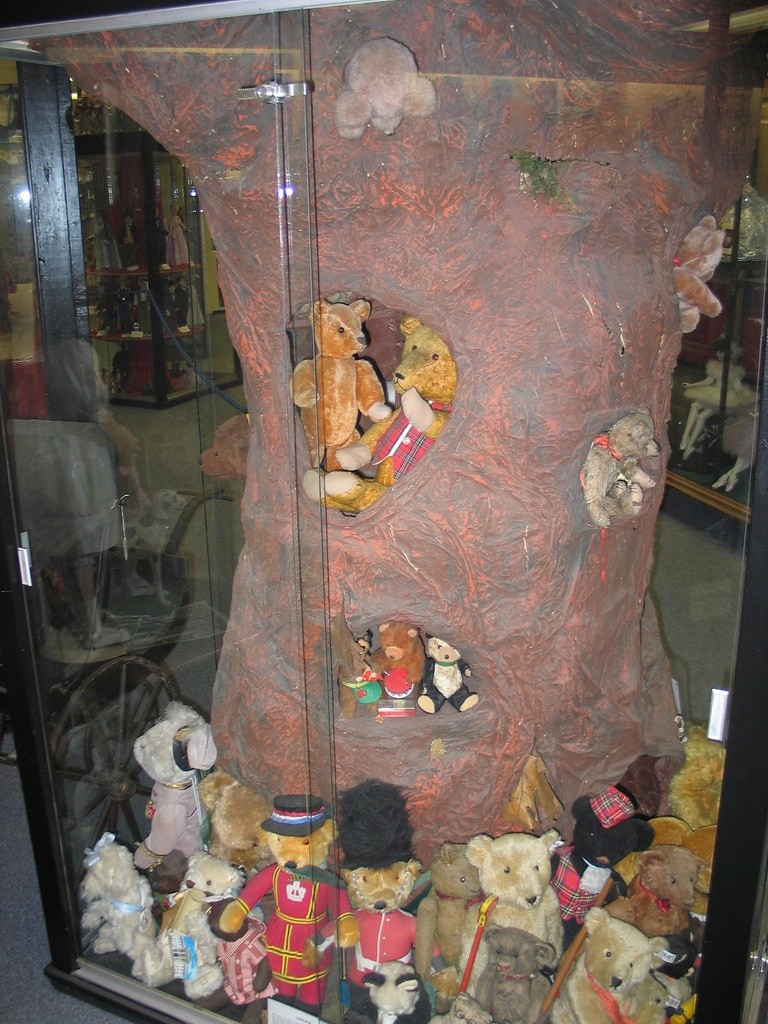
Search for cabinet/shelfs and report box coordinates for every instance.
[73,131,242,408]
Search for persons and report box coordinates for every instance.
[40,340,133,649]
[89,203,206,397]
[680,338,759,491]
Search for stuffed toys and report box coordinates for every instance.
[334,37,435,138]
[199,414,251,484]
[78,622,724,1024]
[580,406,662,529]
[673,216,724,332]
[302,315,455,512]
[290,299,394,473]
